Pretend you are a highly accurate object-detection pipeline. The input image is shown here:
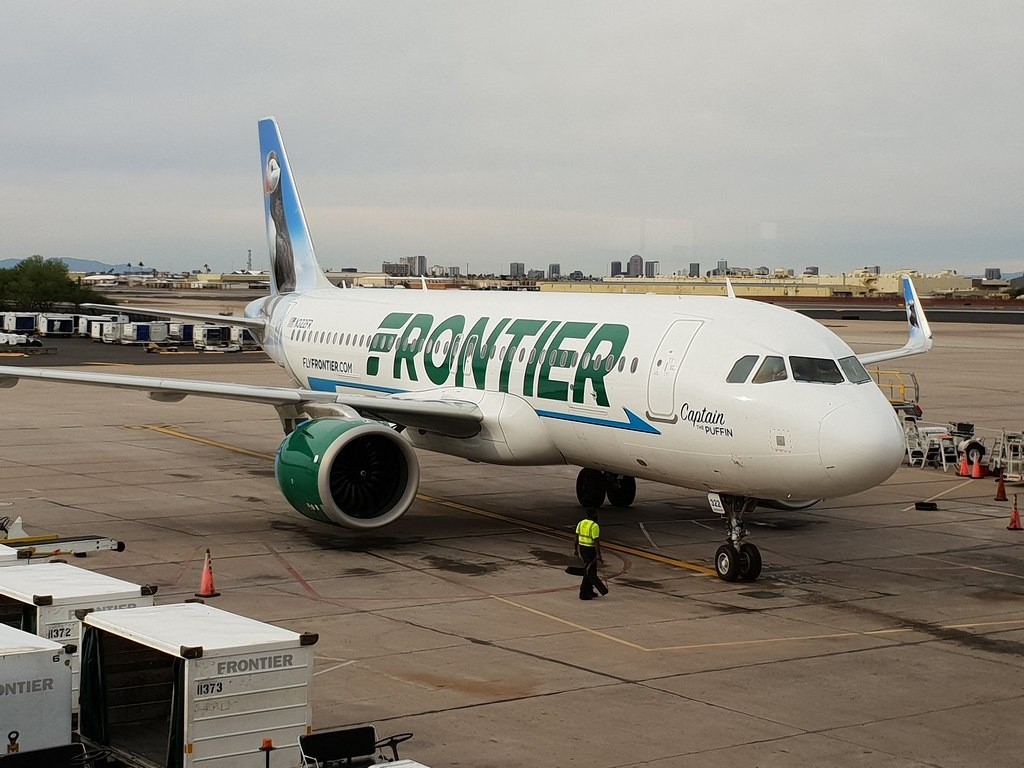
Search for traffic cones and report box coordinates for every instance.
[969,455,984,479]
[994,470,1009,501]
[1006,509,1023,530]
[956,459,971,477]
[195,548,221,599]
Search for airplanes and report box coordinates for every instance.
[0,114,935,585]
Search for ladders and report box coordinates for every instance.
[902,416,923,467]
[920,436,959,472]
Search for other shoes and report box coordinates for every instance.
[580,592,598,600]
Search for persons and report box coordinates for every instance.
[573,506,609,602]
